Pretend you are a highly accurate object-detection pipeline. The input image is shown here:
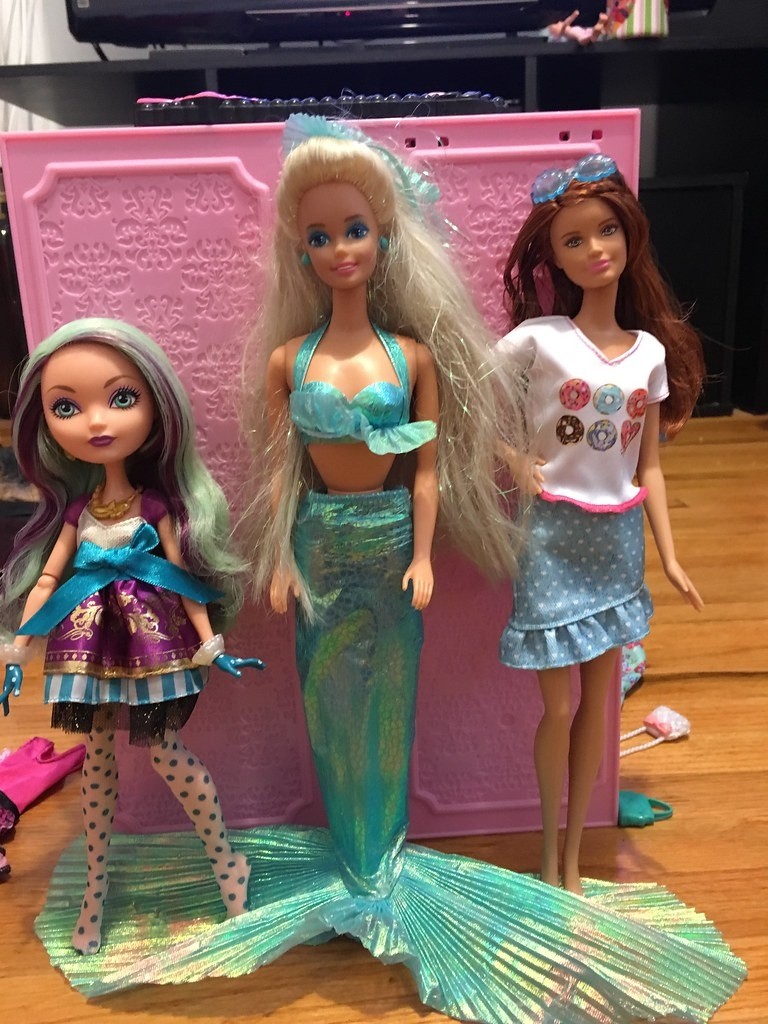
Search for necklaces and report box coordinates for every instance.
[88,481,141,518]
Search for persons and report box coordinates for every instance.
[0,319,267,957]
[33,113,749,1023]
[484,154,704,899]
